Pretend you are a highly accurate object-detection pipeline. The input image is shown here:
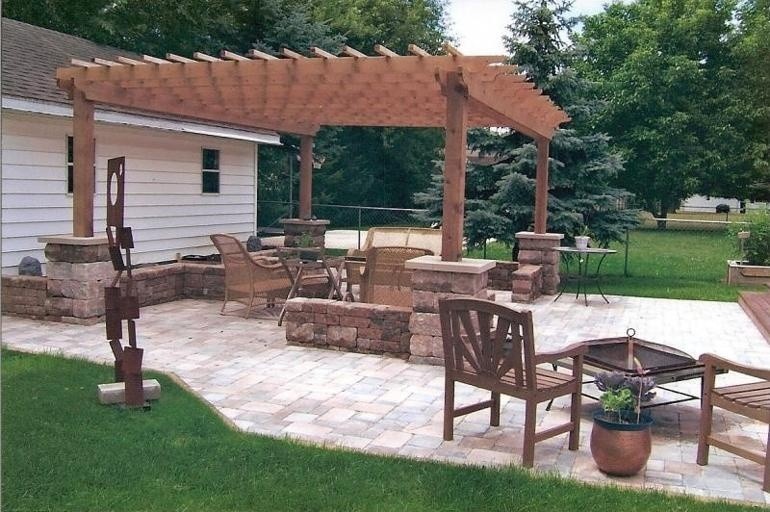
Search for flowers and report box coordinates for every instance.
[595,357,656,423]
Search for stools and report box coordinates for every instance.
[697,352,769,493]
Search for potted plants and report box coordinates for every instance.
[726,205,770,285]
[575,223,590,250]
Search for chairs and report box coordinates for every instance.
[438,295,590,468]
[209,234,435,327]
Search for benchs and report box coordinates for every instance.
[346,226,444,291]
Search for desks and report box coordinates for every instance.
[550,245,617,307]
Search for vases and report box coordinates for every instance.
[590,412,654,478]
